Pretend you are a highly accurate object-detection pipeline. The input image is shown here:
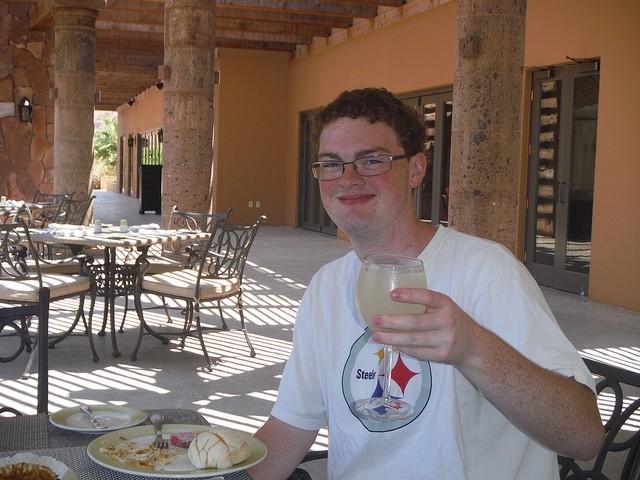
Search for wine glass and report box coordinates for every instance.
[350,252,430,423]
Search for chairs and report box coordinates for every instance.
[27,190,69,257]
[0,218,100,380]
[118,205,234,335]
[287,358,640,480]
[42,195,96,261]
[130,216,268,371]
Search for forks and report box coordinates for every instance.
[150,415,167,449]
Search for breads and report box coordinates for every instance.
[187,428,253,470]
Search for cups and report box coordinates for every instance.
[94,220,102,232]
[120,219,128,232]
[1,196,6,204]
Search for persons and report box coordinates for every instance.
[245,88,607,478]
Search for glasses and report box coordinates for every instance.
[308,153,410,182]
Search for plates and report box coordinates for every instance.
[0,452,78,480]
[86,424,267,477]
[49,405,148,430]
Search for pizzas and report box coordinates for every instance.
[1,461,61,479]
